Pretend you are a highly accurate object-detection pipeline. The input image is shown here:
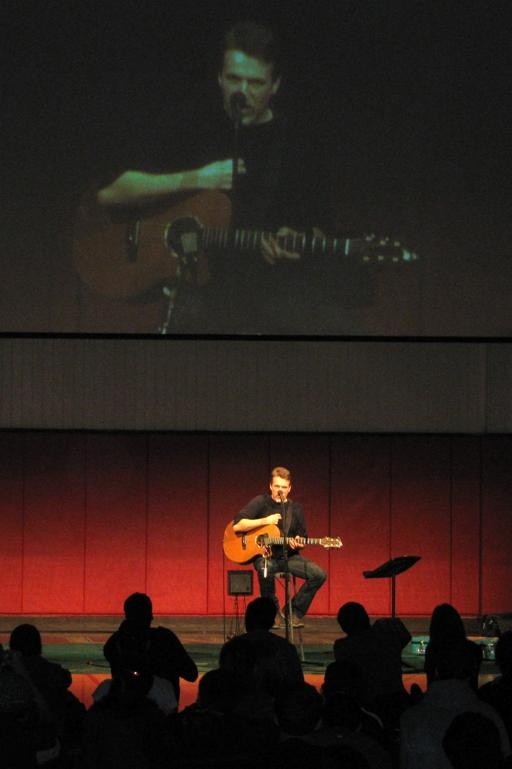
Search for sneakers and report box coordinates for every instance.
[272,604,305,629]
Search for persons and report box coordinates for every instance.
[231,466,329,628]
[93,22,375,315]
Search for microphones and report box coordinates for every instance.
[229,91,246,132]
[278,490,284,503]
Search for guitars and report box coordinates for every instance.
[224,519,343,565]
[75,188,417,298]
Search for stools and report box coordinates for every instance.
[270,573,306,666]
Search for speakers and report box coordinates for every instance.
[228,570,253,595]
